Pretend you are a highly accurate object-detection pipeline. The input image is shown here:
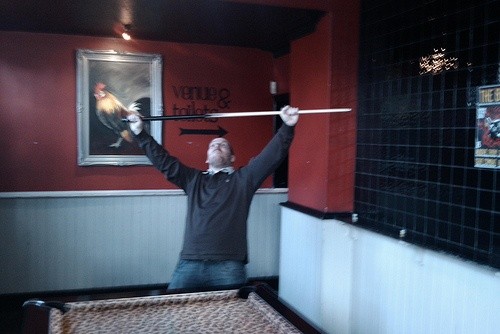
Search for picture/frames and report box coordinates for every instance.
[76,49,163,166]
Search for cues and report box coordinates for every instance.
[120,108,352,123]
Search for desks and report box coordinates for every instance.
[20,282,319,334]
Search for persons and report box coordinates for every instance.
[125,105,299,295]
